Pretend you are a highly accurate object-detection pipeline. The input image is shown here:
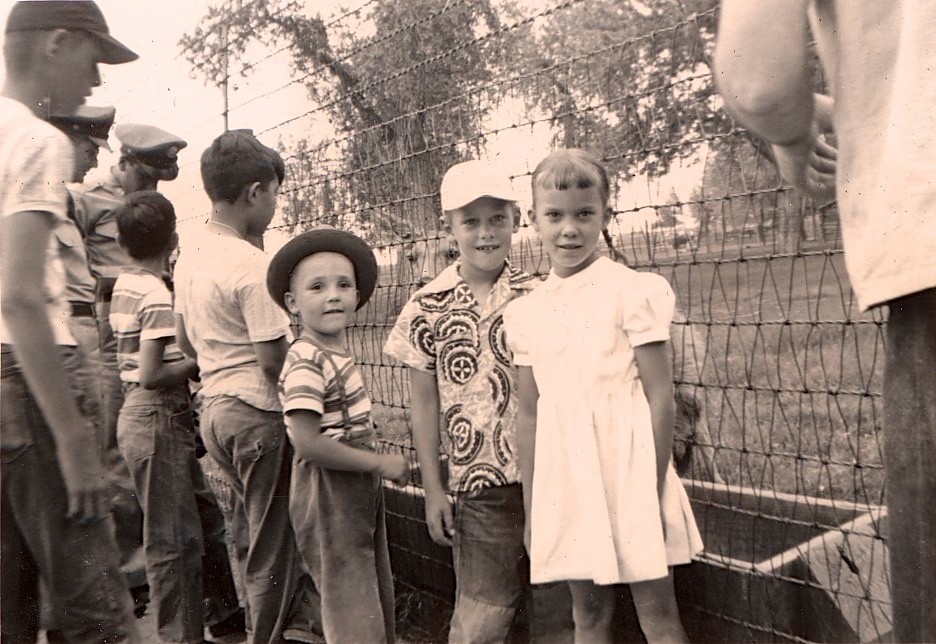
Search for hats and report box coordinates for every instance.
[5,1,139,65]
[440,159,518,211]
[115,123,187,181]
[42,105,116,153]
[266,224,378,316]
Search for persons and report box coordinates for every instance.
[385,161,576,643]
[504,148,704,644]
[176,129,329,644]
[707,0,936,644]
[53,102,243,644]
[1,0,144,644]
[265,225,410,644]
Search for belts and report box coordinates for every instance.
[70,302,97,317]
[102,290,112,302]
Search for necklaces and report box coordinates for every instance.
[209,222,245,239]
[126,267,159,277]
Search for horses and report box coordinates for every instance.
[374,212,725,484]
